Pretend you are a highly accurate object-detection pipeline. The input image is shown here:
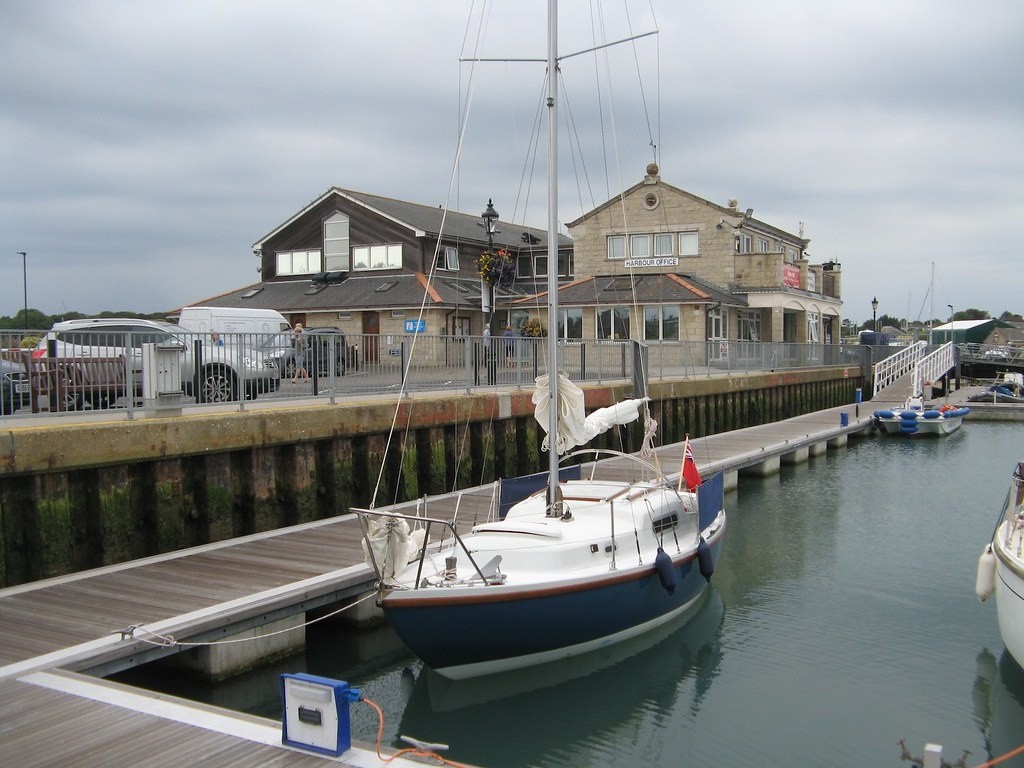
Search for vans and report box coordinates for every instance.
[176,305,298,355]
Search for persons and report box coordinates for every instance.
[482,323,491,369]
[503,327,516,366]
[292,323,310,384]
[212,332,224,347]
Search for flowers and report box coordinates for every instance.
[472,249,515,287]
[521,320,547,341]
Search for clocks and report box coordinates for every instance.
[646,196,657,207]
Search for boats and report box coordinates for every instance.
[975,460,1024,672]
[875,402,970,438]
[966,381,1024,403]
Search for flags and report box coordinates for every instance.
[682,440,701,494]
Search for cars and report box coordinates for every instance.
[0,356,32,416]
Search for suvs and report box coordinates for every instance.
[256,325,353,378]
[33,318,282,411]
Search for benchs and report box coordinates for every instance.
[22,353,144,413]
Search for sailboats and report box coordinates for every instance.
[346,1,728,683]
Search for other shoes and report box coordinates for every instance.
[292,379,297,383]
[304,379,311,383]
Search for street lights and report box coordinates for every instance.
[16,250,29,331]
[479,197,499,382]
[871,297,879,331]
